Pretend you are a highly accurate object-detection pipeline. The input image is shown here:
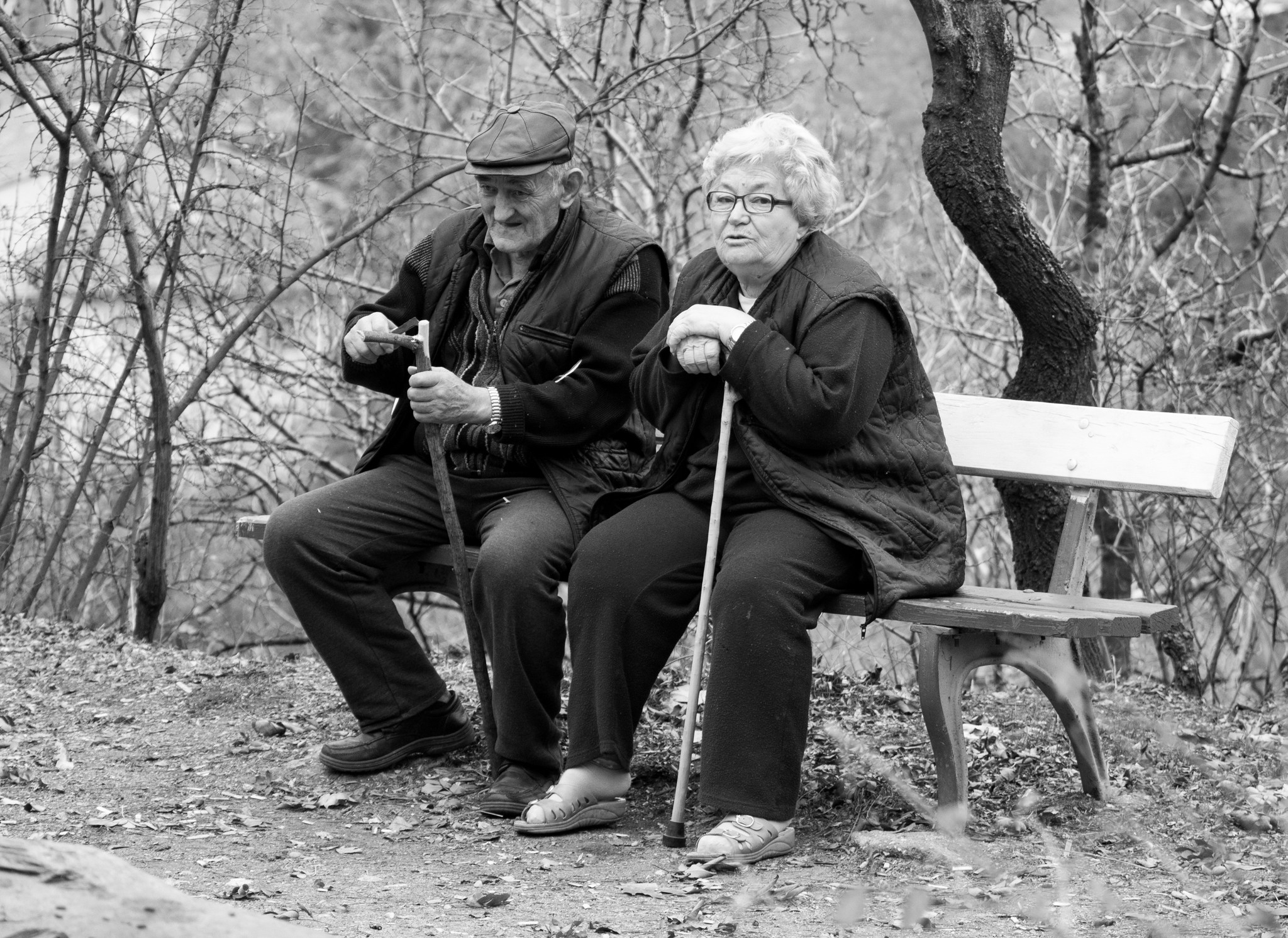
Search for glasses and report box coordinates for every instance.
[706,191,793,213]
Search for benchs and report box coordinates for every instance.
[233,390,1244,838]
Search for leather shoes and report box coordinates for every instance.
[480,767,554,814]
[319,690,474,772]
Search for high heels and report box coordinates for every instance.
[513,784,626,833]
[687,811,796,863]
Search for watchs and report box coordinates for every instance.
[727,320,761,351]
[481,384,502,435]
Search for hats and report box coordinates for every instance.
[464,100,576,177]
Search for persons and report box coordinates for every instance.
[507,112,967,871]
[262,108,678,821]
